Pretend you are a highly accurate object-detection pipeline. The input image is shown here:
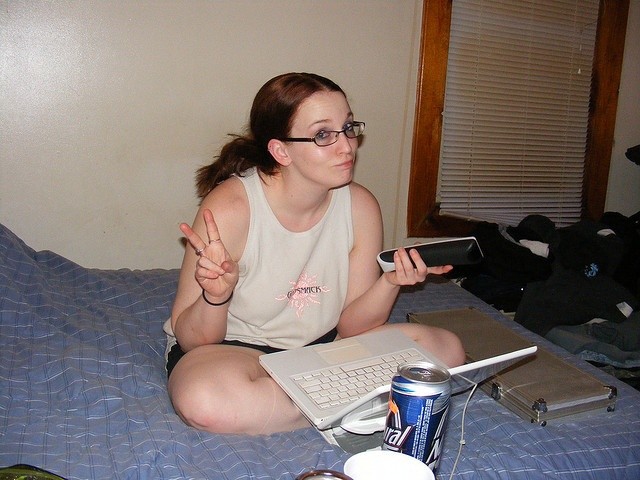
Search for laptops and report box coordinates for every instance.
[258,327,537,430]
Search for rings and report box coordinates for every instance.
[195,243,207,256]
[207,239,221,244]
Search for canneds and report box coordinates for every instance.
[381,361,452,479]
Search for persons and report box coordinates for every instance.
[162,71,466,435]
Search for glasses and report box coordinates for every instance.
[284,123,365,145]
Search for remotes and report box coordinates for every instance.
[377,237,482,274]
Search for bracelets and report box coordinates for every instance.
[201,287,234,307]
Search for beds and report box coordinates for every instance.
[2,224,639,480]
